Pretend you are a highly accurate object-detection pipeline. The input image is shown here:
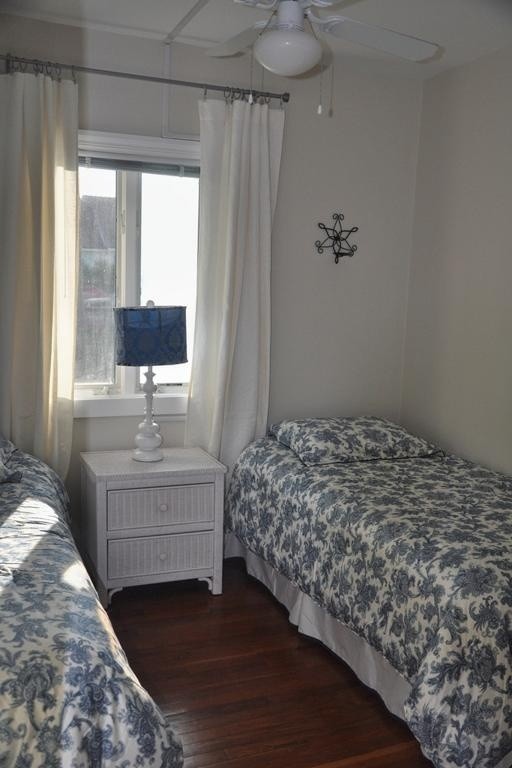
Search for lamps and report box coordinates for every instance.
[253,0,324,77]
[114,302,189,462]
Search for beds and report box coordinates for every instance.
[1,451,183,768]
[227,435,511,767]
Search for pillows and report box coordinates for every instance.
[0,434,23,484]
[271,414,442,467]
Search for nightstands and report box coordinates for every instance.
[79,445,228,609]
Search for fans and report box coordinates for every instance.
[203,0,440,74]
